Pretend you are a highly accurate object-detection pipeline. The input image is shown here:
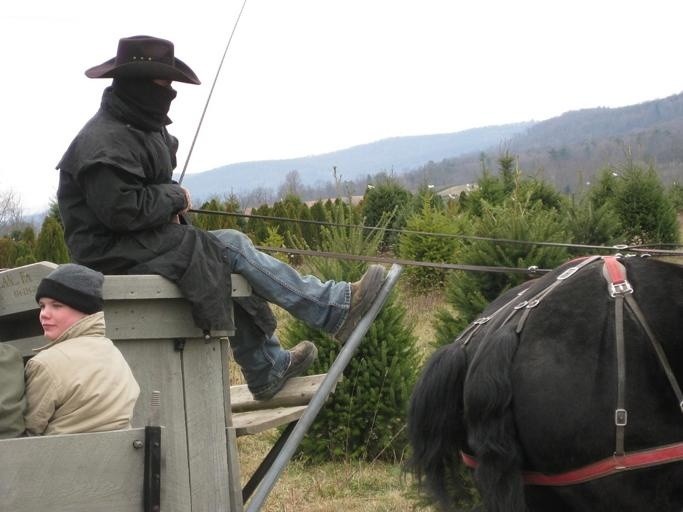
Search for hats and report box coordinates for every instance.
[84,34,201,86]
[35,263,104,315]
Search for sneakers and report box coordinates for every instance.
[331,263,386,349]
[250,341,318,401]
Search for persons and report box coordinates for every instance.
[19,263,139,435]
[53,36,390,400]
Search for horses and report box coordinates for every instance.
[459,253,683,511]
[397,275,544,512]
